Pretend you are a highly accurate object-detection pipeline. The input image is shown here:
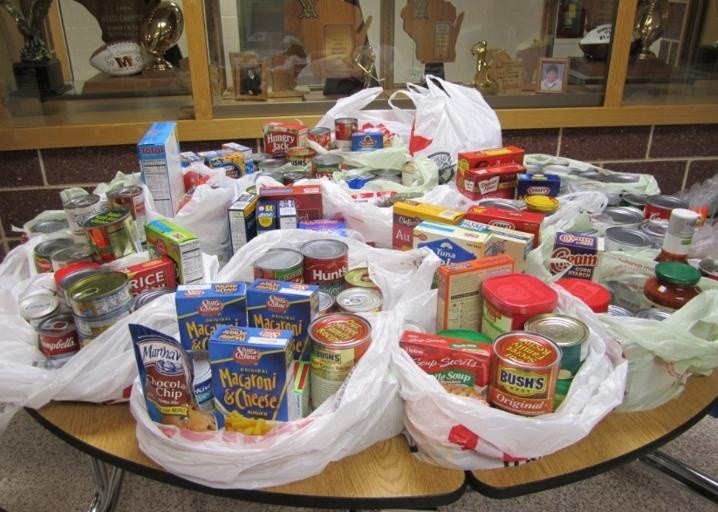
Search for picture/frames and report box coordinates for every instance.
[235,57,269,101]
[535,57,571,95]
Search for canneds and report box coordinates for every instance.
[19,118,718,417]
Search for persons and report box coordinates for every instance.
[242,68,261,96]
[471,40,493,85]
[540,65,562,91]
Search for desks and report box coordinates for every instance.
[1,148,718,511]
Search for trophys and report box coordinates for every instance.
[629,0,673,63]
[139,1,184,75]
[0,0,74,96]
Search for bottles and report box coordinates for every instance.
[642,207,701,311]
